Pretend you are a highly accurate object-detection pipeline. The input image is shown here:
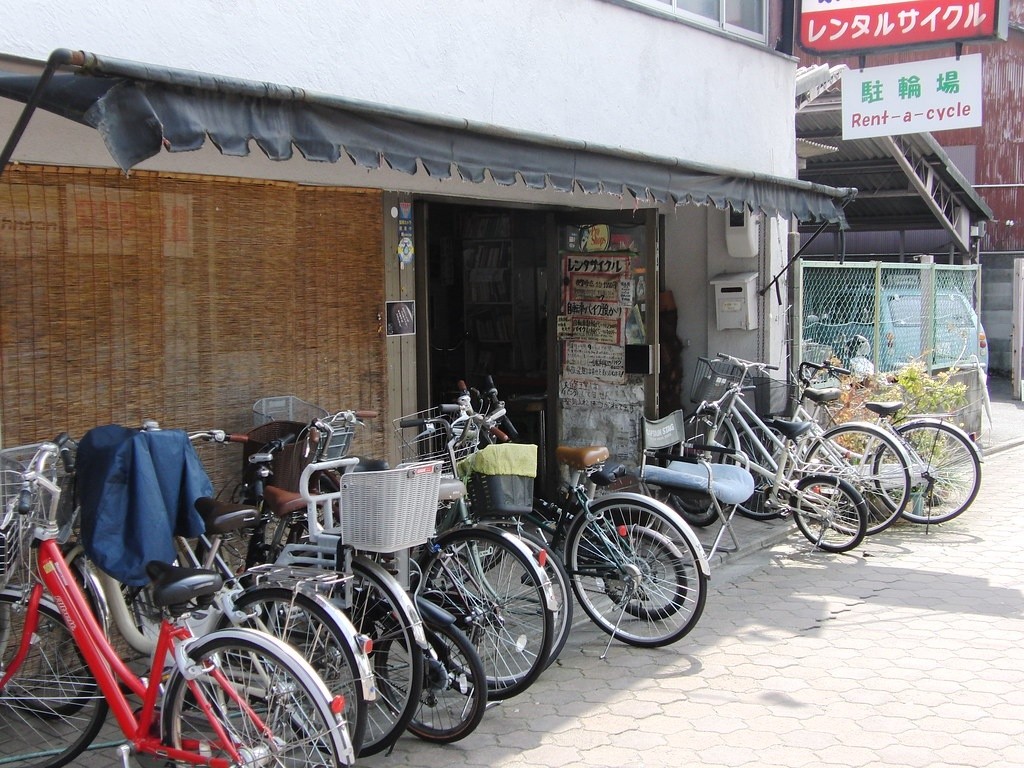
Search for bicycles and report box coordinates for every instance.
[0,440,356,768]
[0,353,984,767]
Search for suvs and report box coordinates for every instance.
[805,280,990,404]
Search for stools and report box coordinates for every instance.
[504,392,548,483]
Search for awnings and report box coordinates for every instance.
[0,48,857,230]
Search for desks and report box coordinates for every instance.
[478,370,547,393]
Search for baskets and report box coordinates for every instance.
[0,468,39,591]
[458,443,538,518]
[689,357,753,412]
[392,406,480,481]
[241,419,320,493]
[0,443,66,523]
[752,377,800,417]
[252,395,330,427]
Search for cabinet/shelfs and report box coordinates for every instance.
[459,205,536,369]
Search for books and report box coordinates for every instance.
[462,216,513,343]
